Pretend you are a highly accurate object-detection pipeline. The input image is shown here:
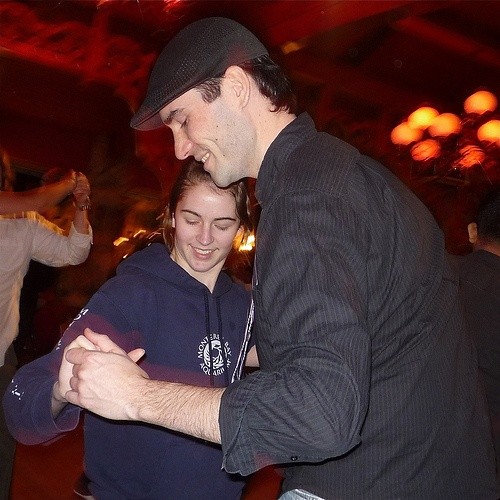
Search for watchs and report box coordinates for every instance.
[73,196,91,211]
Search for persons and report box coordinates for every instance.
[2,158,262,500]
[0,169,78,216]
[452,188,500,490]
[0,151,94,497]
[66,16,500,500]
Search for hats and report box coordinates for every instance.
[130,17,269,131]
